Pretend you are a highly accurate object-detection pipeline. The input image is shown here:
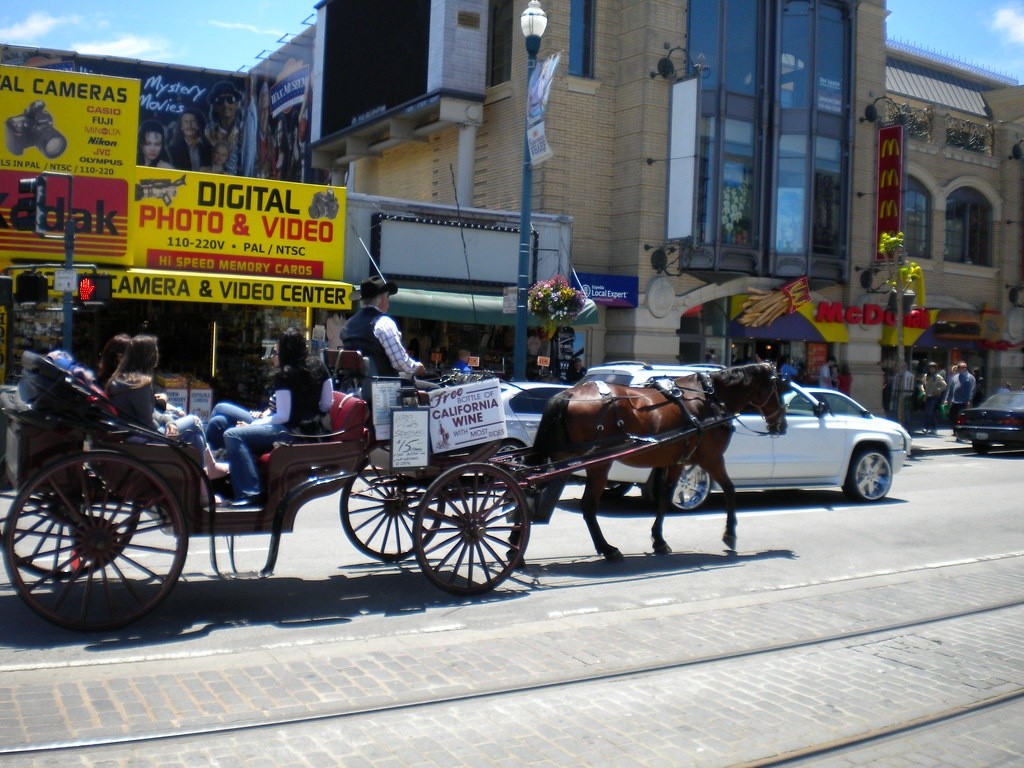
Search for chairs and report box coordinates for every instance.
[324,346,431,410]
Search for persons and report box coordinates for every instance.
[139,82,243,175]
[102,335,231,507]
[246,38,316,182]
[570,358,587,383]
[781,355,799,380]
[755,352,765,363]
[889,363,915,424]
[452,351,473,374]
[819,357,840,390]
[1000,382,1011,393]
[205,331,333,507]
[920,360,984,443]
[705,354,717,364]
[339,276,441,391]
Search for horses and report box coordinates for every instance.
[506,353,788,568]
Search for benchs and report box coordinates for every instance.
[259,390,371,502]
[88,383,201,509]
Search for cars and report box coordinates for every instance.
[467,379,576,481]
[952,389,1024,457]
[779,385,913,459]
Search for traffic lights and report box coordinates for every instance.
[16,175,47,234]
[77,272,113,303]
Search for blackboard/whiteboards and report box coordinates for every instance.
[390,406,430,472]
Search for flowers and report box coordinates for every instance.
[530,277,586,337]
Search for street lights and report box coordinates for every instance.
[508,0,550,381]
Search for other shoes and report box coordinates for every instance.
[229,494,263,508]
[924,429,937,437]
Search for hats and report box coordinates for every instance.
[347,275,398,301]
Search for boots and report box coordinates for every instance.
[203,444,233,480]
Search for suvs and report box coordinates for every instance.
[563,357,909,513]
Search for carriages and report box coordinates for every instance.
[1,345,790,636]
